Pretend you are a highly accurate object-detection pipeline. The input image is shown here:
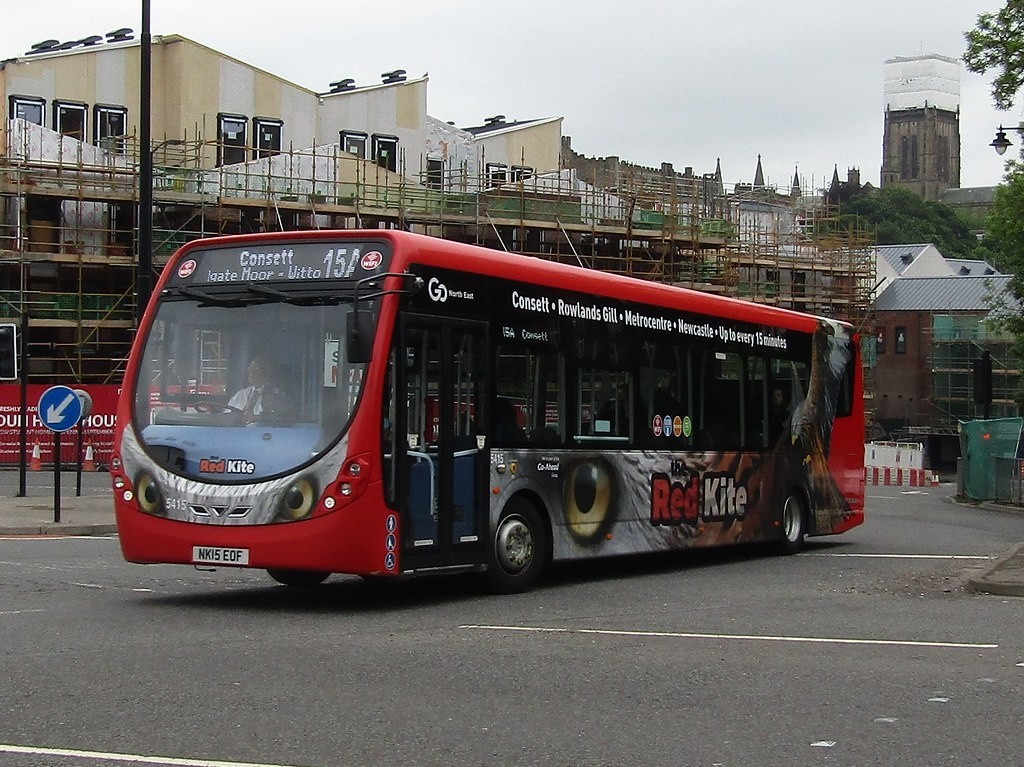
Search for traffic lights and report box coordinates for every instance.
[973,359,992,404]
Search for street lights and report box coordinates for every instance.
[138,139,185,433]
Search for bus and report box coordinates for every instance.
[111,229,865,594]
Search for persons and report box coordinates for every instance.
[599,386,629,434]
[768,389,789,445]
[223,358,290,426]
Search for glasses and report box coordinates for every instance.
[246,365,265,372]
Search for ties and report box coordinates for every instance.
[243,391,257,414]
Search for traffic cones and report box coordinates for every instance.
[26,436,46,471]
[81,437,97,472]
